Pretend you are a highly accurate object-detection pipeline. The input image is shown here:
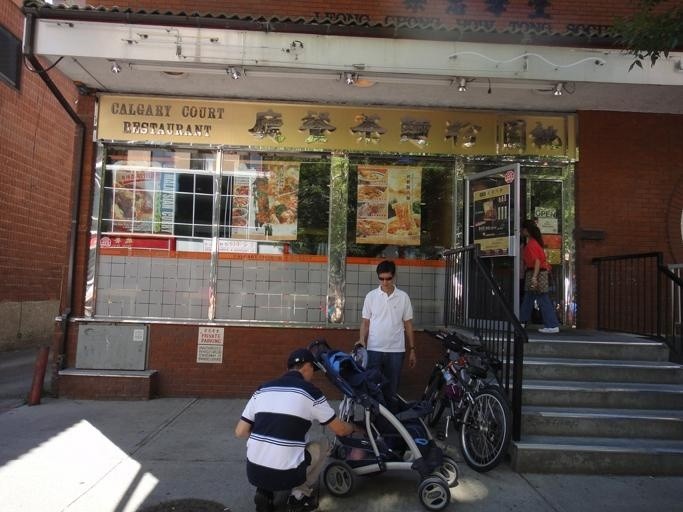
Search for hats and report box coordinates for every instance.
[288,348,320,373]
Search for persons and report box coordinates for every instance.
[354,260,417,393]
[233,347,366,511]
[520,219,560,333]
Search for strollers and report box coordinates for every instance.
[305,340,459,511]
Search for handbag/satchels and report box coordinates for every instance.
[524,268,549,294]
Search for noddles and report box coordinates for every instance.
[388,201,420,236]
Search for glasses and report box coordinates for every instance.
[378,275,394,281]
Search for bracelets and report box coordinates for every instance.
[351,422,356,434]
[409,346,416,350]
[531,277,537,279]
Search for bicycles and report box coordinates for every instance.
[424,329,511,472]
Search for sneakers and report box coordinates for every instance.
[254,488,274,511]
[538,327,560,334]
[287,494,318,511]
